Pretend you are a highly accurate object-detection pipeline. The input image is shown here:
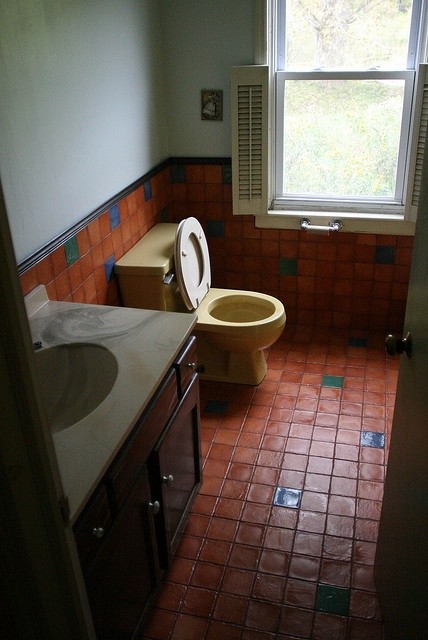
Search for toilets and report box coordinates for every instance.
[115,217,286,384]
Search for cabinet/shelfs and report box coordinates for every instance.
[74,334,203,640]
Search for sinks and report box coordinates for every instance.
[30,343,123,435]
